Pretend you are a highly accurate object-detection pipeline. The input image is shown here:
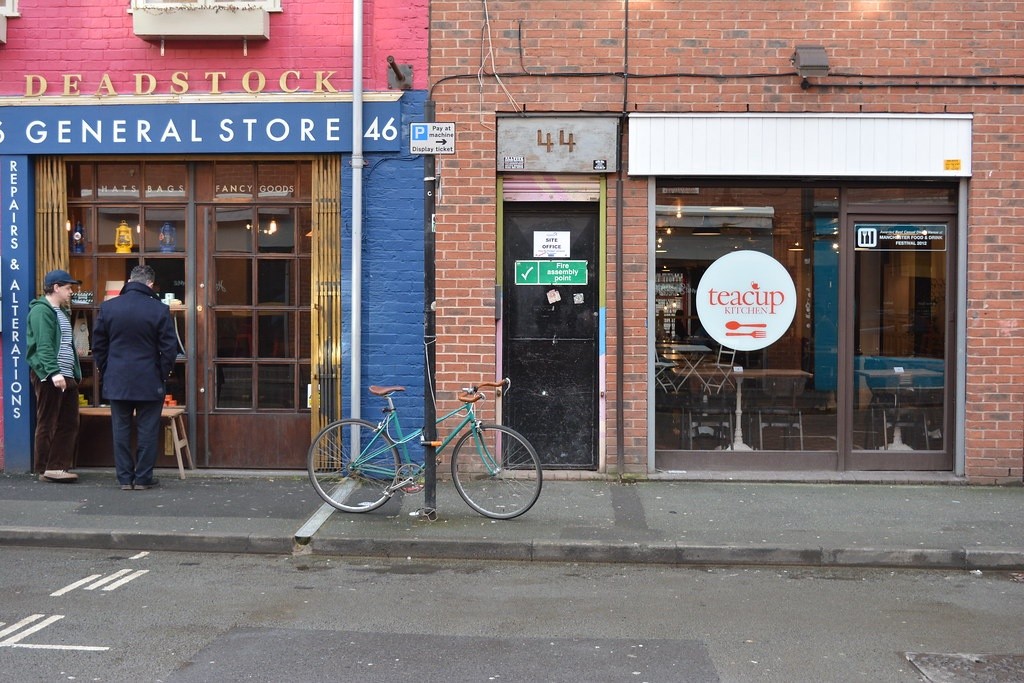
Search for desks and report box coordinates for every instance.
[79,407,194,480]
[671,345,712,393]
[671,368,814,448]
[855,369,943,450]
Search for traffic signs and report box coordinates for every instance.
[409,122,456,155]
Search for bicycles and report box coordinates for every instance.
[305,376,543,521]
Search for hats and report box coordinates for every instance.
[45,270,78,285]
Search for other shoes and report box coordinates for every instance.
[134,477,160,490]
[39,470,79,483]
[121,484,133,490]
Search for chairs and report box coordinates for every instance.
[655,348,677,394]
[748,375,805,451]
[703,344,737,393]
[679,375,734,450]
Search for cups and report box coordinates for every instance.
[165,293,175,305]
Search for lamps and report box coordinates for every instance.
[789,43,829,78]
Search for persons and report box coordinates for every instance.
[25,270,83,483]
[92,264,178,490]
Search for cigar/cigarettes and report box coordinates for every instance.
[62,388,65,393]
